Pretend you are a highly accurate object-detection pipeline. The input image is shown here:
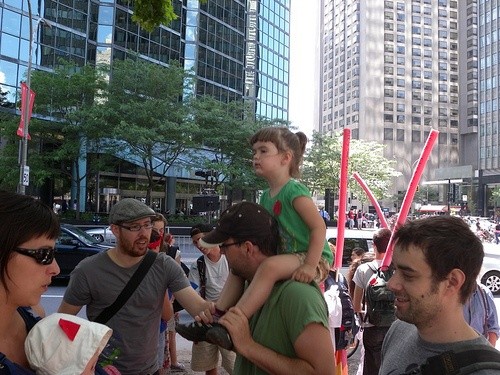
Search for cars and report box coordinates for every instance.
[359,211,500,245]
[29,223,124,287]
[472,242,500,296]
[303,225,388,297]
[83,225,121,243]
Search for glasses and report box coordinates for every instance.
[116,221,154,231]
[13,246,59,265]
[218,239,257,255]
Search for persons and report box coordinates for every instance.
[1,192,128,374]
[350,227,399,374]
[196,199,339,374]
[173,126,335,350]
[183,223,238,374]
[145,209,185,375]
[315,241,355,374]
[315,204,500,314]
[377,212,499,375]
[57,195,215,374]
[458,276,500,349]
[23,312,115,375]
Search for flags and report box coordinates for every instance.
[17,79,36,140]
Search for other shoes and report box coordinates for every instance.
[173,319,235,353]
[170,362,186,372]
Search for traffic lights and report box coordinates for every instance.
[454,183,460,200]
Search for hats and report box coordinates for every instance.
[190,223,214,238]
[108,198,157,224]
[198,201,279,248]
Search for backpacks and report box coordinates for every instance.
[325,273,361,351]
[364,261,397,327]
